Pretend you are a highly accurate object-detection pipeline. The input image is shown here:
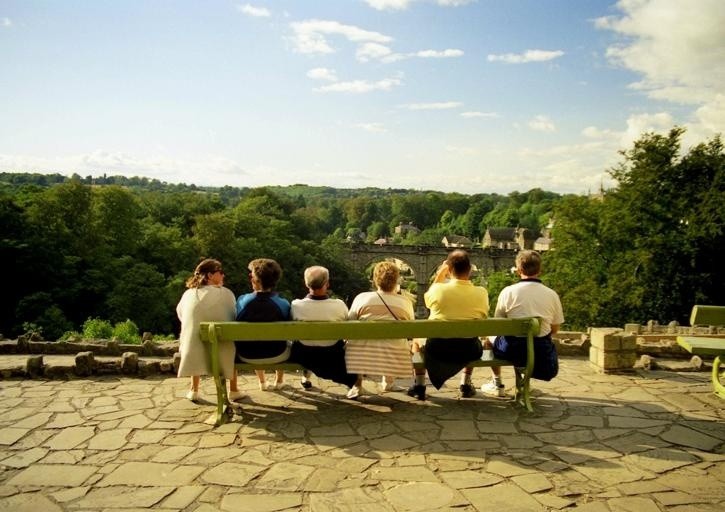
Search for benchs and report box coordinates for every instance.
[198,316,542,427]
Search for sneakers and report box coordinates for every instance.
[481,383,506,398]
[300,379,312,391]
[186,388,199,401]
[346,385,365,399]
[258,379,287,391]
[381,380,408,393]
[405,384,426,400]
[229,389,247,400]
[459,383,478,398]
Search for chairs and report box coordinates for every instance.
[675,303,725,401]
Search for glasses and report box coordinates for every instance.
[214,268,224,276]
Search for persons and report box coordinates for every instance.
[480,248,565,399]
[288,266,362,397]
[175,258,247,401]
[346,260,417,399]
[407,247,490,402]
[233,258,293,390]
